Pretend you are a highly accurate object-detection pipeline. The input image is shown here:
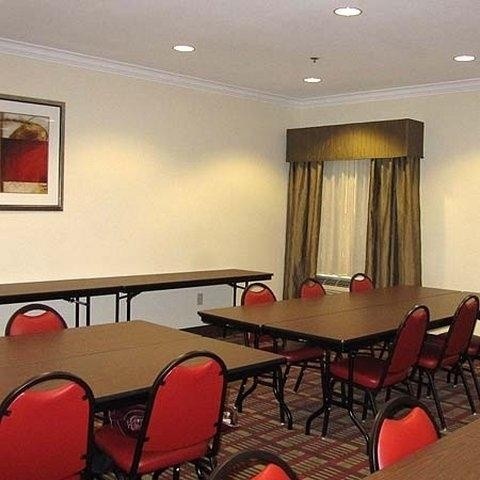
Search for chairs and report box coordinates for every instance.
[0,299,232,480]
[210,272,479,480]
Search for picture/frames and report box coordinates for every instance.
[0,90,66,213]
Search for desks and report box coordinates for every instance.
[0,319,288,480]
[198,282,477,434]
[0,267,274,321]
